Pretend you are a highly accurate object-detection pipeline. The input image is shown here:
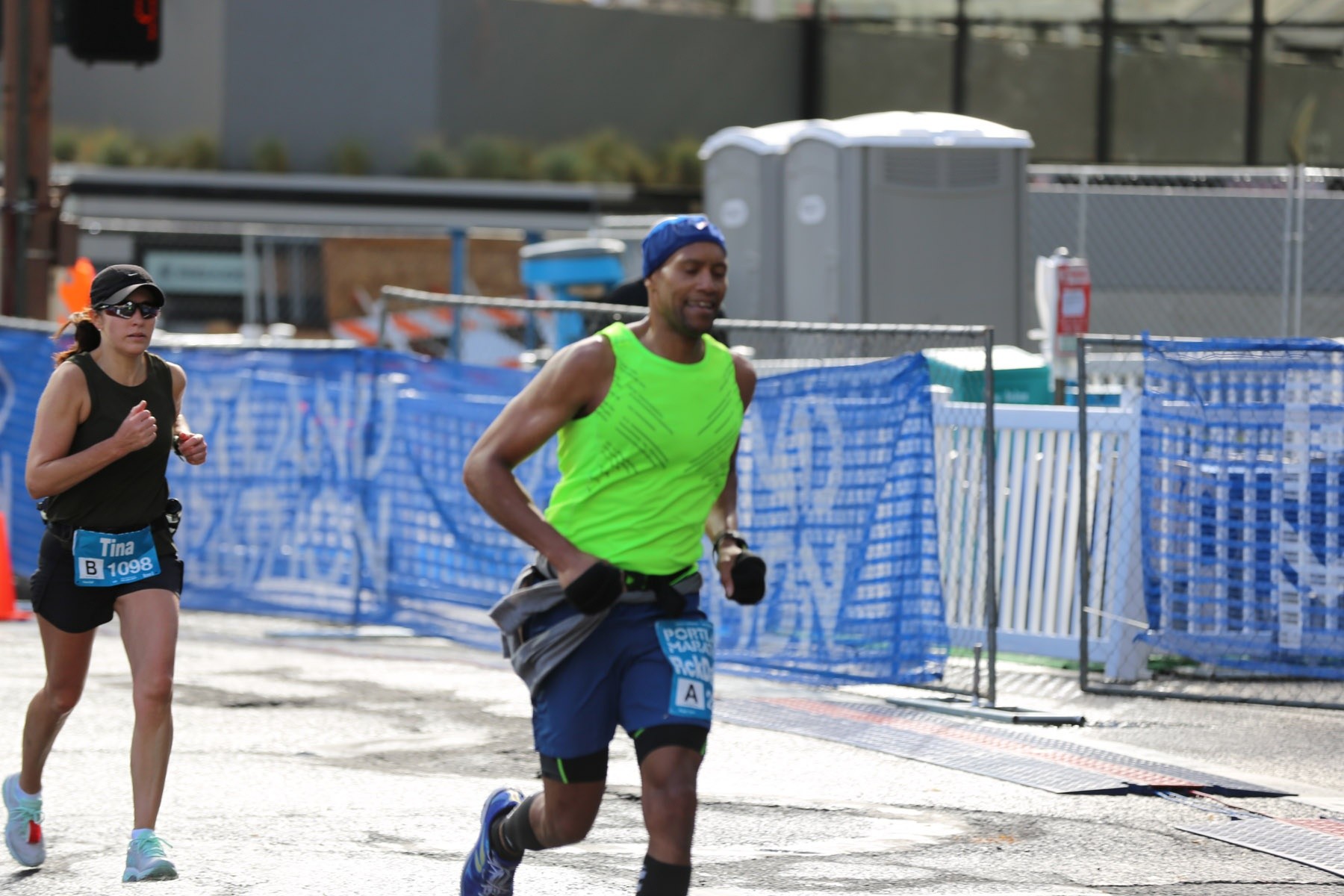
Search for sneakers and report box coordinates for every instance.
[122,833,178,882]
[2,772,45,867]
[460,785,526,896]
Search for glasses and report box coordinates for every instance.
[97,299,160,319]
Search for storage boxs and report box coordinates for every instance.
[922,344,1050,406]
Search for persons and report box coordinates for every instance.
[459,216,767,896]
[2,261,208,882]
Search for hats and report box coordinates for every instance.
[642,215,728,276]
[90,264,165,310]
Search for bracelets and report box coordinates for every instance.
[173,434,184,462]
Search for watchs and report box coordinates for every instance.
[711,531,749,565]
